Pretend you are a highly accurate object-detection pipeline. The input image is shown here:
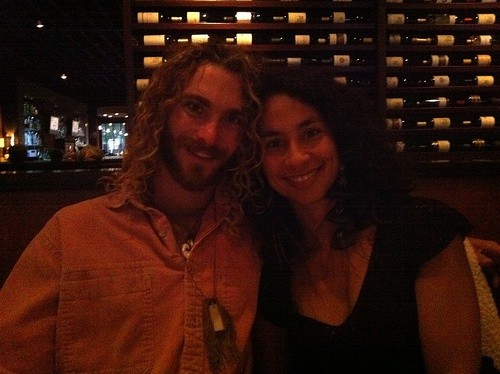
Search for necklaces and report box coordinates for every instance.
[175,195,245,374]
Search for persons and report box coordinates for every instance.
[0,46,500,374]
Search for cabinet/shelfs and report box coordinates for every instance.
[122,0,500,178]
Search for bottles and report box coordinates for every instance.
[385,54,496,66]
[386,33,497,45]
[143,33,374,45]
[335,76,371,86]
[136,77,150,92]
[136,10,368,24]
[386,12,496,24]
[386,75,497,87]
[261,55,368,66]
[394,139,500,152]
[386,94,500,107]
[142,55,169,68]
[384,115,496,127]
